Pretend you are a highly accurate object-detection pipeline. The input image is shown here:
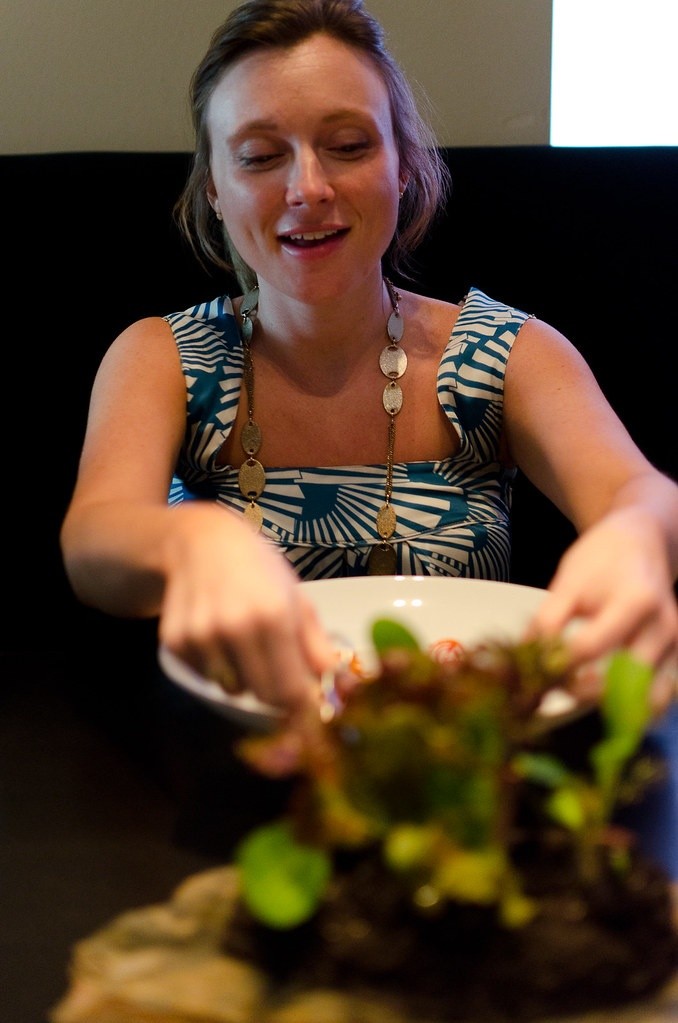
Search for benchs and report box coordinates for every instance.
[0,140,678,609]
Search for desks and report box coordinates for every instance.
[0,550,677,1023]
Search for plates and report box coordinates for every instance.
[159,577,614,743]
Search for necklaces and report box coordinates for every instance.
[239,275,407,579]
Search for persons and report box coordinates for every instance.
[60,0,678,713]
